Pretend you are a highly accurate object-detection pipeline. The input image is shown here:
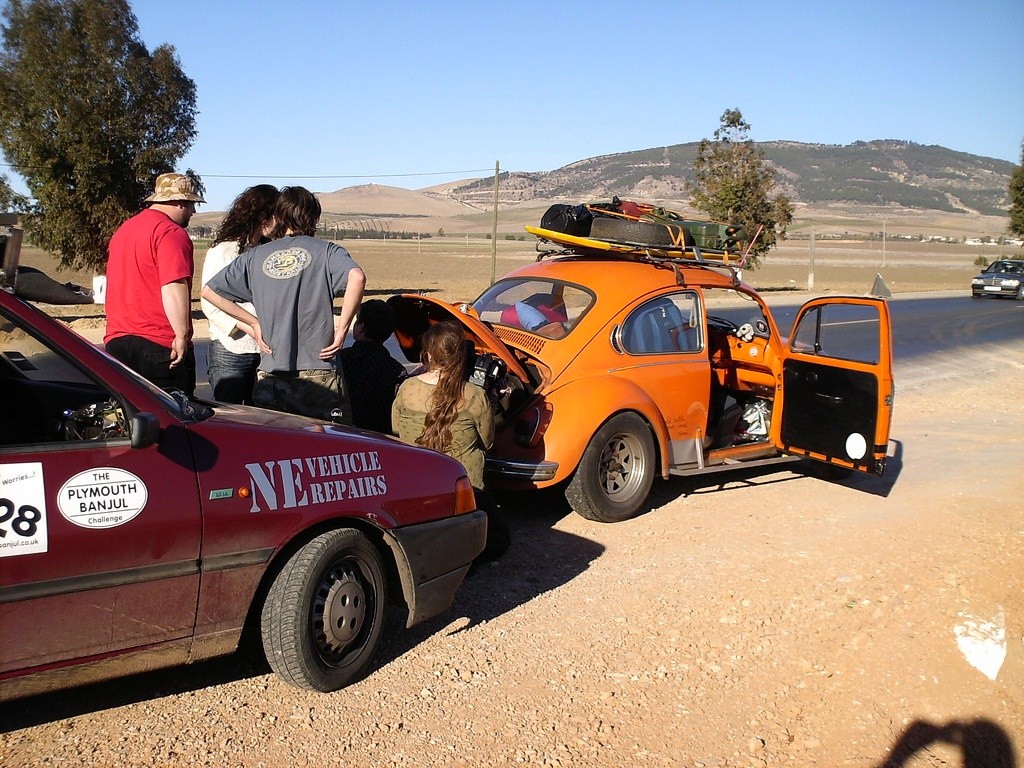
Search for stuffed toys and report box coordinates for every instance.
[737,323,754,342]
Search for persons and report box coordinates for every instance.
[1016,264,1024,273]
[391,321,495,493]
[202,185,367,421]
[997,264,1007,273]
[200,184,279,406]
[339,298,407,434]
[103,173,207,396]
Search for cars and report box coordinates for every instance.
[383,204,896,523]
[972,259,1024,300]
[0,286,488,701]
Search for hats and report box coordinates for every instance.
[143,173,207,204]
